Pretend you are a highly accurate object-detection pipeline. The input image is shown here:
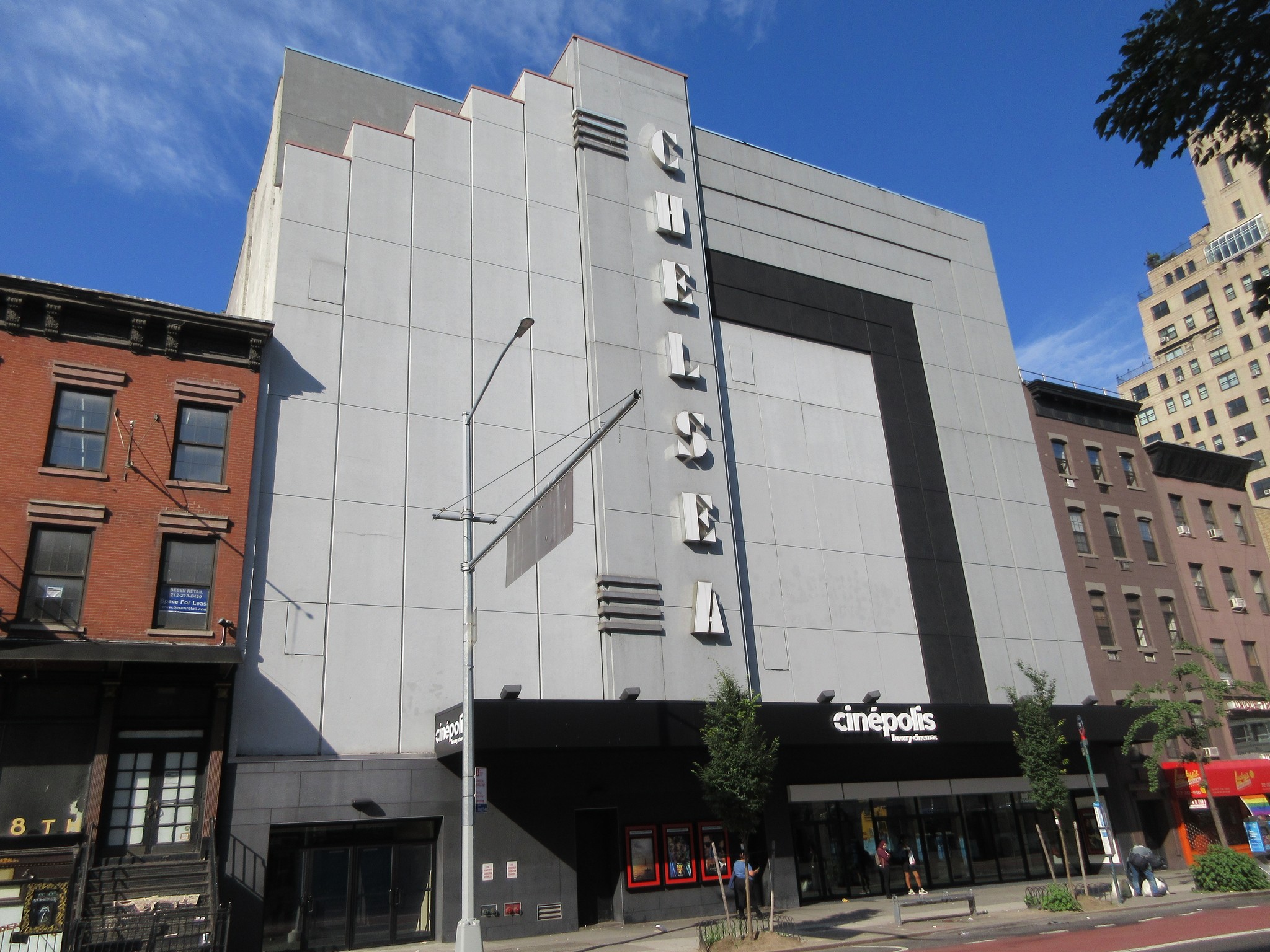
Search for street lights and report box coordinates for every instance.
[455,317,536,952]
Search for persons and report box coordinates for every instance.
[702,834,727,875]
[877,841,898,899]
[851,842,873,895]
[900,839,928,895]
[1128,845,1163,897]
[732,852,767,919]
[668,835,691,878]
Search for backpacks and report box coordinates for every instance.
[875,848,884,868]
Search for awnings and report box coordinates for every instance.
[1162,759,1270,799]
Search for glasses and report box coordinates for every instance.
[704,842,711,848]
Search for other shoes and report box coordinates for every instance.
[1135,893,1144,897]
[1153,893,1163,897]
[739,914,746,920]
[860,891,867,894]
[757,911,764,920]
[918,888,929,895]
[908,888,917,896]
[867,890,871,893]
[886,894,898,899]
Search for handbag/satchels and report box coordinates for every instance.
[728,871,736,890]
[1131,854,1145,866]
[908,851,916,865]
